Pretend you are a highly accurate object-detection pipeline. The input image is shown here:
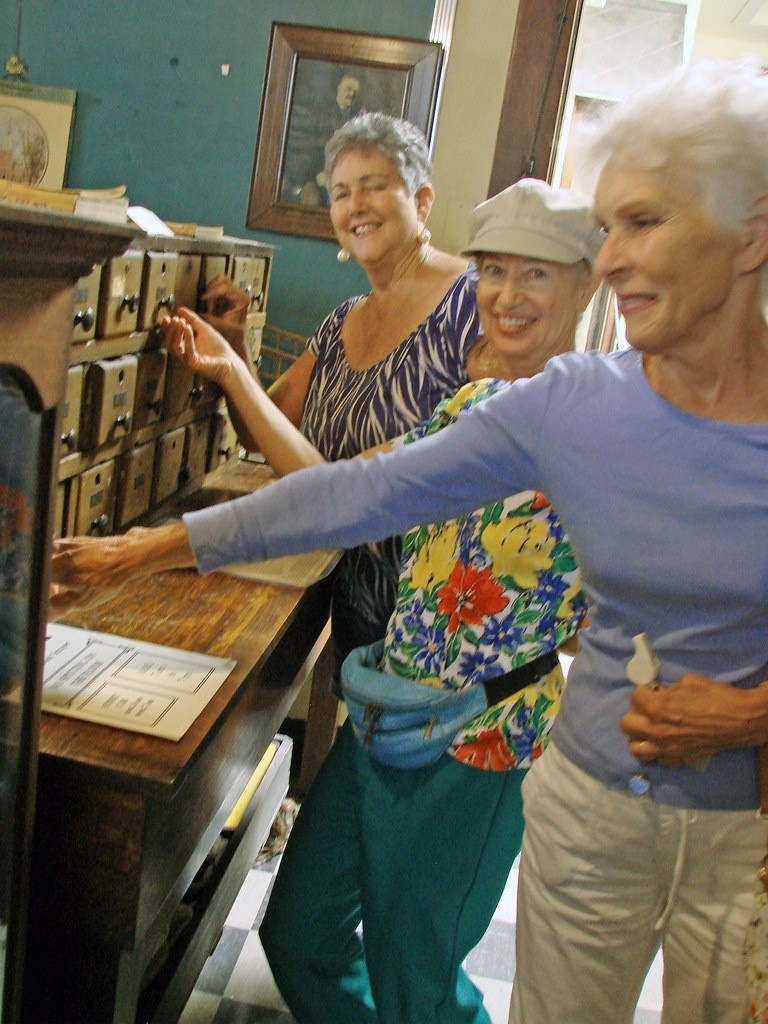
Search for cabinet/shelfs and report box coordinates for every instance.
[21,460,344,1024]
[50,222,275,552]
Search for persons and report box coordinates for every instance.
[297,64,370,209]
[48,49,768,1024]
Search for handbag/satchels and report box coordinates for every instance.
[338,639,561,770]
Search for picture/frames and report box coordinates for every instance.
[245,19,444,245]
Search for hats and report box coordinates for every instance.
[459,177,606,266]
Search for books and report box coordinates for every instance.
[162,215,225,241]
[0,181,129,225]
